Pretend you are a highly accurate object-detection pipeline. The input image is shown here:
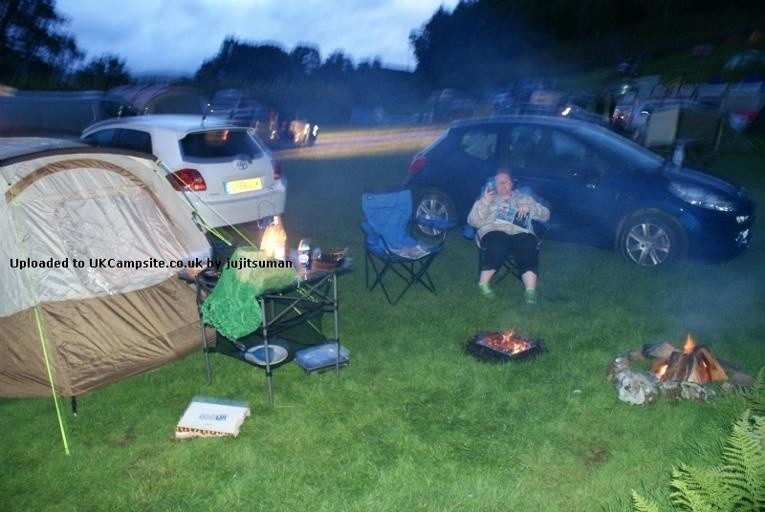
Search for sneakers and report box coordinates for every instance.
[525,288,539,306]
[477,282,496,301]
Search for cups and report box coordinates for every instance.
[296,243,312,272]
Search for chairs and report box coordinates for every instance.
[461,176,552,286]
[357,184,458,305]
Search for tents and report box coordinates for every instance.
[0,136,216,400]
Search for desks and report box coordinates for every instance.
[182,247,355,407]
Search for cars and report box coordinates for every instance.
[77,113,288,230]
[409,115,756,266]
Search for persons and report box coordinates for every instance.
[255,111,319,148]
[466,168,550,304]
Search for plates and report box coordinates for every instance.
[245,343,288,366]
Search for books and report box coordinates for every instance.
[385,246,430,260]
[496,206,532,233]
